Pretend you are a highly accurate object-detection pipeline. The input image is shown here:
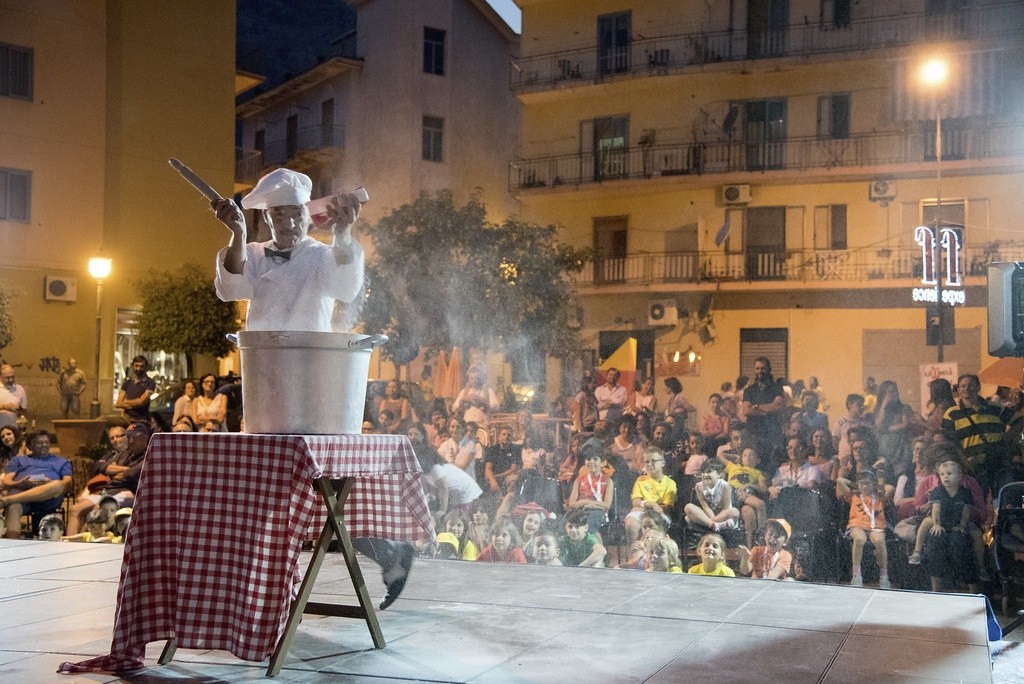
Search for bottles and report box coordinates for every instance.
[90,398,100,419]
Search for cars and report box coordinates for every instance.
[150,377,243,424]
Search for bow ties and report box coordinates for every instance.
[264,247,292,261]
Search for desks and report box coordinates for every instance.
[131,433,440,674]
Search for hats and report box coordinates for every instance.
[241,168,313,209]
[435,532,459,555]
[765,518,792,540]
[114,507,132,519]
[100,493,120,509]
[512,500,557,520]
[122,423,148,437]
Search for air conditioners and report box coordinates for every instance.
[649,299,679,327]
[653,150,693,171]
[871,181,896,202]
[721,185,750,203]
[556,307,580,329]
[44,275,77,302]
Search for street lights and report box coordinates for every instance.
[88,248,113,420]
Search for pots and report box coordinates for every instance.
[226,330,389,436]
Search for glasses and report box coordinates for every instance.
[852,446,867,453]
[643,458,663,464]
[786,445,807,452]
[202,379,215,385]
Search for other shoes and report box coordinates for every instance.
[908,552,921,565]
[880,576,891,589]
[794,563,808,581]
[851,575,863,586]
[715,519,734,532]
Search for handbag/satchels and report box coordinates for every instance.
[986,442,1006,472]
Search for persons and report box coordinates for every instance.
[0,355,244,545]
[360,355,1024,619]
[210,168,365,332]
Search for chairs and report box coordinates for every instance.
[2,459,77,536]
[474,413,908,588]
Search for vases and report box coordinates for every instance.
[52,419,109,458]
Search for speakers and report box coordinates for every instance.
[985,260,1024,358]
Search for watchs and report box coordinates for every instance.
[754,403,759,412]
